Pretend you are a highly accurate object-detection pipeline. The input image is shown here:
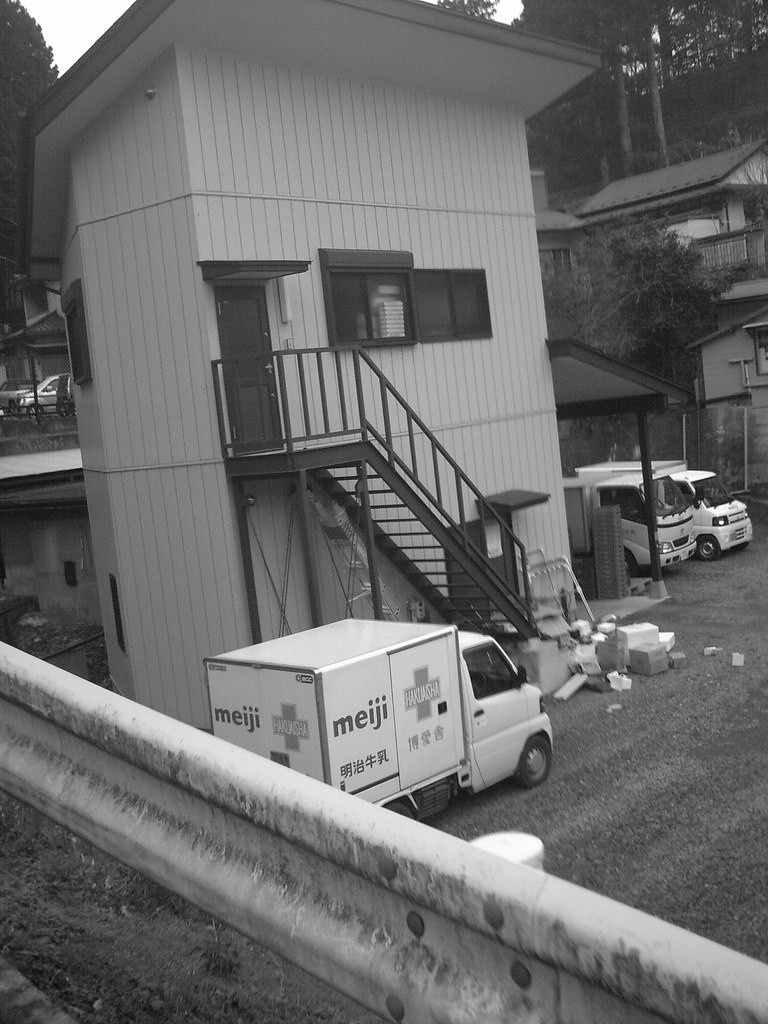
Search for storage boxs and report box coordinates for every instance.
[617,621,668,677]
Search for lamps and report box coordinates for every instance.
[146,90,155,99]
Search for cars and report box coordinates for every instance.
[18,373,69,419]
[1,378,44,414]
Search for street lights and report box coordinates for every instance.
[683,413,691,462]
[741,406,747,491]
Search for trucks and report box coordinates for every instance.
[575,461,754,564]
[201,615,553,820]
[563,473,705,574]
